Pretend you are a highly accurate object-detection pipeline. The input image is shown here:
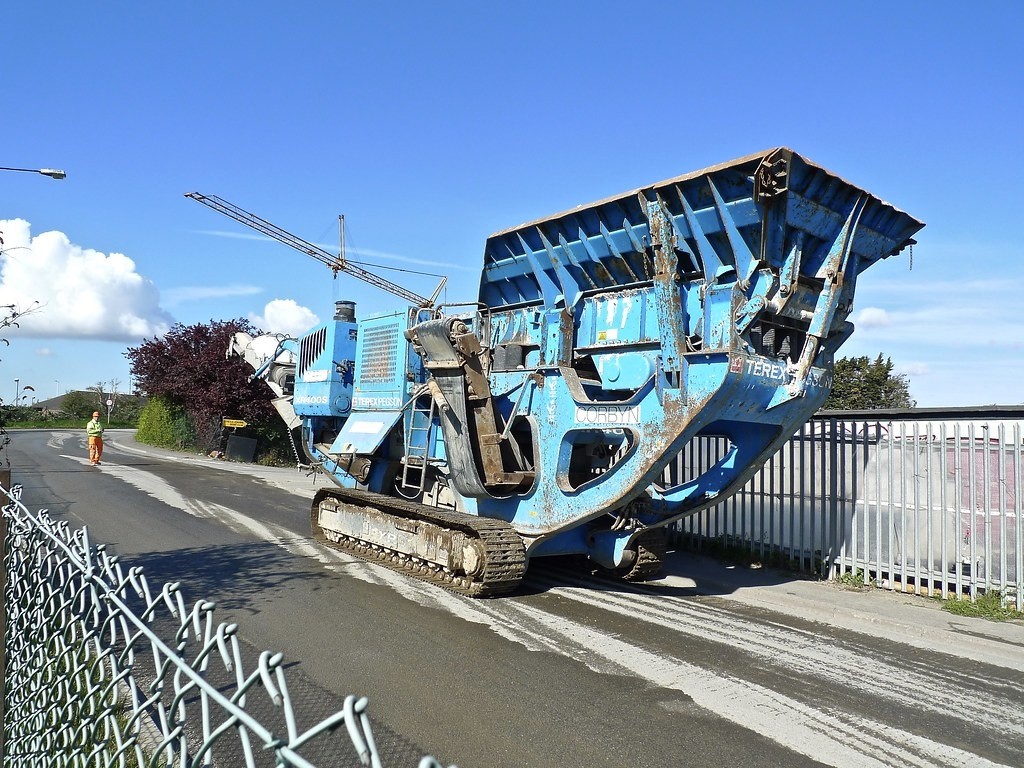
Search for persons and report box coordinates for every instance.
[86,412,105,465]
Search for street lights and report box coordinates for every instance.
[1,163,66,181]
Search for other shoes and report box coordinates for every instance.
[91,463,96,466]
[96,463,101,465]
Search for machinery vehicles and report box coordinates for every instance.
[180,142,926,604]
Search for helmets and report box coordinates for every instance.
[92,412,100,417]
